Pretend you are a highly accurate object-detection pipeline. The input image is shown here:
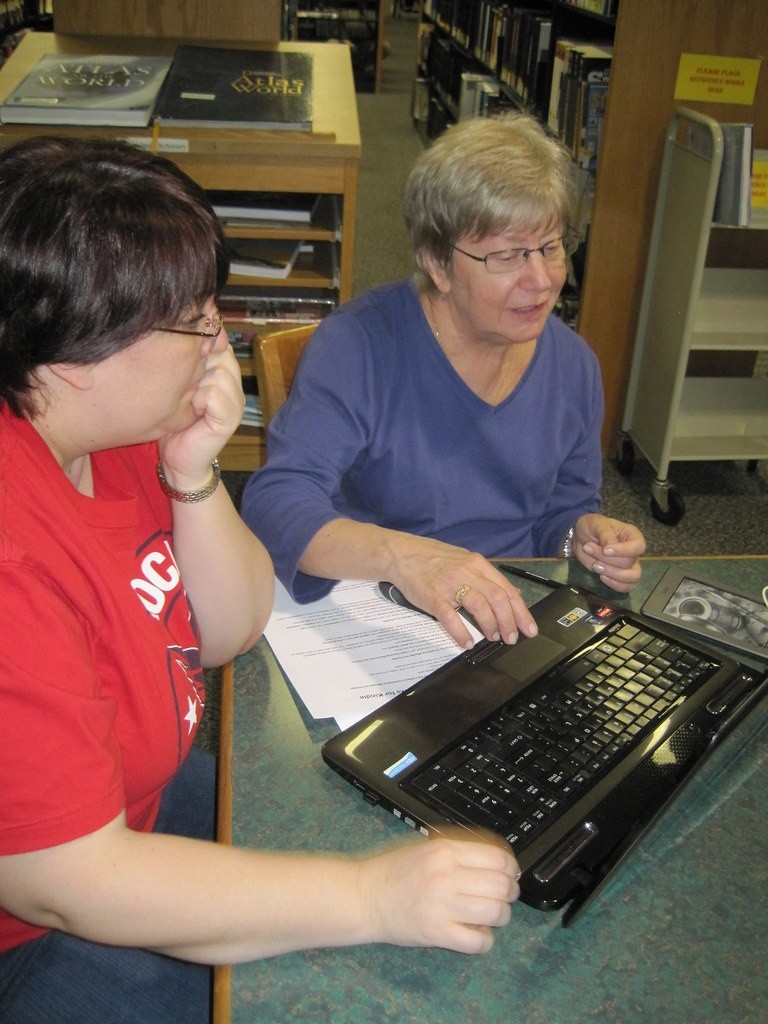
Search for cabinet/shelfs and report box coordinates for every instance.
[0,0,768,523]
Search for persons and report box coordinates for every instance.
[241,109,647,650]
[0,136,522,1023]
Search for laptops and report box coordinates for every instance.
[319,584,768,931]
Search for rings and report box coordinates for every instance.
[455,585,469,605]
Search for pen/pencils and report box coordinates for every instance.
[499,563,564,589]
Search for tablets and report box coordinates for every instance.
[639,564,768,668]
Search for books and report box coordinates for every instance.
[228,240,303,280]
[413,0,621,333]
[216,286,337,428]
[207,191,321,228]
[0,32,314,131]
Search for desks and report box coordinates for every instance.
[220,560,768,1024]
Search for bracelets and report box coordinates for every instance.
[157,456,221,504]
[563,527,574,559]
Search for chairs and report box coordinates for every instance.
[253,321,323,422]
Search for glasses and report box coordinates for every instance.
[148,303,225,353]
[454,222,584,273]
